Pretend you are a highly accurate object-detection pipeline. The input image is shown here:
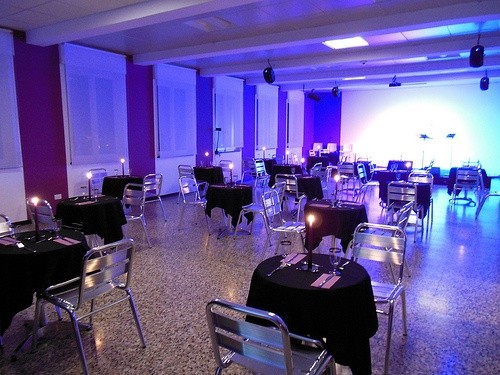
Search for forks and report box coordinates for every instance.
[267,263,286,277]
[338,261,349,270]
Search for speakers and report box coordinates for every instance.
[469,46,484,68]
[263,68,275,84]
[332,87,340,97]
[480,78,489,90]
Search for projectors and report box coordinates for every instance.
[389,82,401,87]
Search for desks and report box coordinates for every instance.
[244,253,378,375]
[102,175,144,210]
[206,183,252,240]
[386,179,430,234]
[377,169,410,202]
[194,165,223,184]
[302,199,370,252]
[447,167,490,197]
[55,195,127,258]
[267,164,301,189]
[0,229,94,336]
[286,174,323,200]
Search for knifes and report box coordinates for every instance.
[284,252,299,263]
[318,275,334,288]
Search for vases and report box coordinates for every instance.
[0,154,493,375]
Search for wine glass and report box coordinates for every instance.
[233,174,238,186]
[280,240,292,267]
[291,167,295,175]
[80,186,87,199]
[328,247,341,276]
[93,189,99,202]
[330,189,342,207]
[396,173,400,182]
[52,219,62,239]
[391,164,398,172]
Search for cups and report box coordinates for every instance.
[223,177,229,184]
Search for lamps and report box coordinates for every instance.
[480,70,489,90]
[332,86,341,97]
[307,90,320,101]
[469,34,485,68]
[263,57,276,83]
